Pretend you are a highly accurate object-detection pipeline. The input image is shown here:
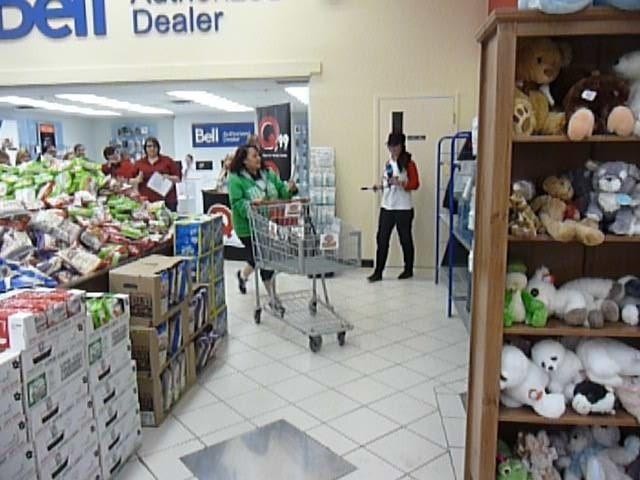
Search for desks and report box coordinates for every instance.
[201,188,248,260]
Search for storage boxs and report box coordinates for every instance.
[0,213,227,479]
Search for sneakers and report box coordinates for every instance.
[237,270,247,295]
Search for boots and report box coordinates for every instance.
[368,270,382,281]
[398,267,413,279]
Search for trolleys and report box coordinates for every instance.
[244,197,361,351]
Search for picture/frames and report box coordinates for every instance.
[38,120,57,158]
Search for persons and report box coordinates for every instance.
[0,138,135,180]
[181,154,196,178]
[229,142,300,315]
[128,136,181,212]
[367,130,421,281]
[216,152,233,191]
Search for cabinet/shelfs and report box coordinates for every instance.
[434,131,472,317]
[464,4,639,480]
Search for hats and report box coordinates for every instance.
[387,133,405,144]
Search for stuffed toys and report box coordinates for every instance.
[499,424,638,478]
[504,264,639,329]
[513,36,639,142]
[499,336,639,420]
[509,155,639,246]
